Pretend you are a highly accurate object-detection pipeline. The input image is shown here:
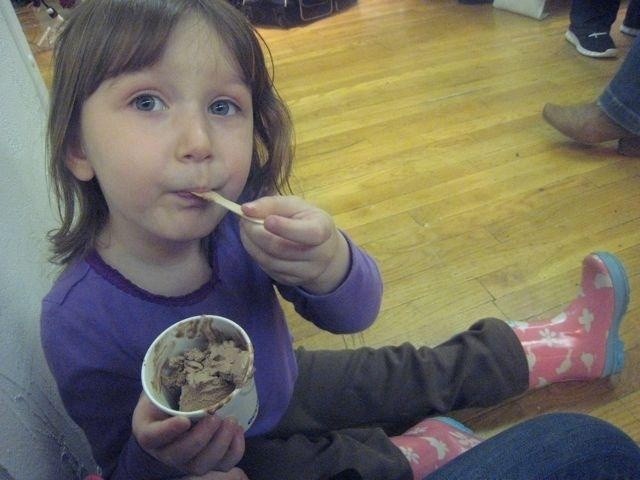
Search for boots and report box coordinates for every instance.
[388,415,482,480]
[510,251,627,392]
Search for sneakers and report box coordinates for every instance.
[619,2,640,37]
[564,22,619,59]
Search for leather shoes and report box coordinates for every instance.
[540,99,640,158]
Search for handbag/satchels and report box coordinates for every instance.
[236,1,356,30]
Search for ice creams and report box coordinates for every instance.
[159,339,249,412]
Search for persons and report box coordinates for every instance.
[539,32,639,161]
[37,1,632,479]
[422,409,638,479]
[563,0,638,59]
